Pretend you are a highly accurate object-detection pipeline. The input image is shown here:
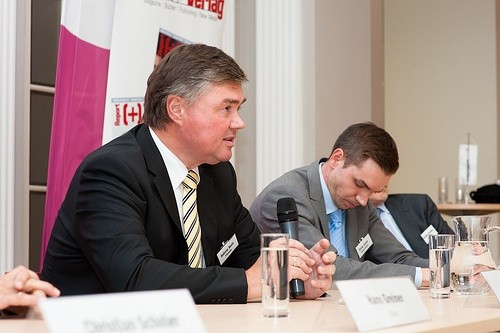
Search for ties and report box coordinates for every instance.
[375,208,383,218]
[329,208,346,257]
[181,171,202,269]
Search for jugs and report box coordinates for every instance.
[451,216,500,295]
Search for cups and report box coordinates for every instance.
[261,234,289,317]
[428,234,454,299]
[456,180,465,204]
[439,176,448,204]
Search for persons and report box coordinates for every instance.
[40,44,336,304]
[247,123,443,289]
[368,185,456,258]
[0,265,61,311]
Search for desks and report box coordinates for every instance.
[0,286,500,333]
[438,204,500,217]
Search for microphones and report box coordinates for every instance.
[277,197,304,296]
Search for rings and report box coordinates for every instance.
[18,291,24,294]
[294,258,297,267]
